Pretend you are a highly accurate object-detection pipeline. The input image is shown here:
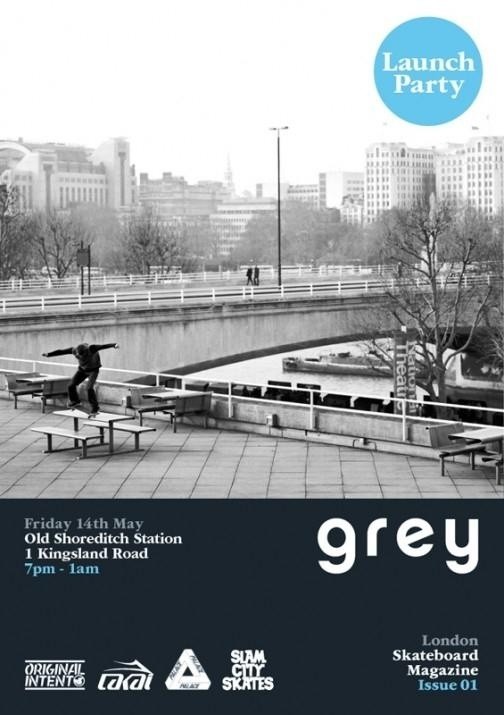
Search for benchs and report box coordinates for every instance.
[427,419,487,457]
[0,365,215,465]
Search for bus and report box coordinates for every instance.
[280,265,312,272]
[320,264,361,274]
[240,263,273,272]
[148,265,182,274]
[79,266,104,274]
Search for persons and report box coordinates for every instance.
[254,264,259,286]
[40,343,120,415]
[246,266,254,286]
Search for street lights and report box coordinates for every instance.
[250,259,253,264]
[268,125,290,283]
[310,258,313,267]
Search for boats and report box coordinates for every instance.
[282,353,446,382]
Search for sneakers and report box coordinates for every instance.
[91,406,99,415]
[66,400,81,409]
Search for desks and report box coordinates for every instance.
[438,425,504,483]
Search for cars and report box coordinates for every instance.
[40,265,58,277]
[453,262,499,276]
